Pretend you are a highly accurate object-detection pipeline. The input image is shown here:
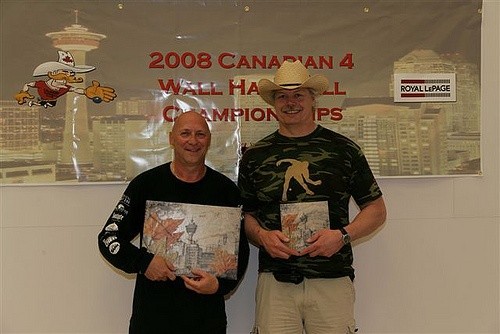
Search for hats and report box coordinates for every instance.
[258,60,330,106]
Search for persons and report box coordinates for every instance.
[237,58,387,334]
[97,111,250,334]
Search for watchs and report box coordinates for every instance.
[339,227,351,245]
